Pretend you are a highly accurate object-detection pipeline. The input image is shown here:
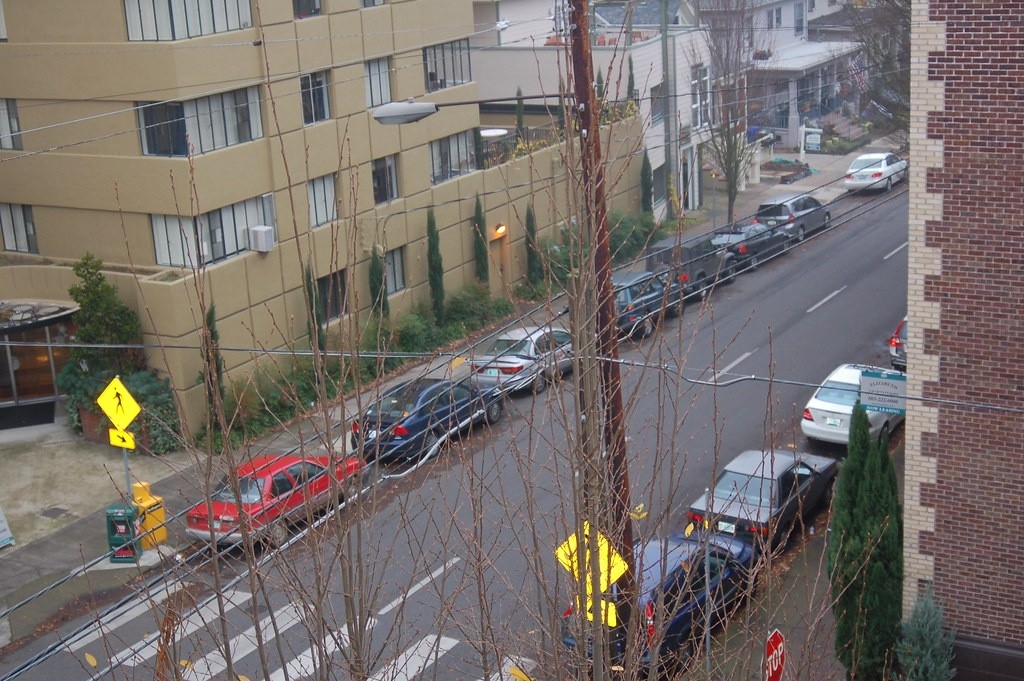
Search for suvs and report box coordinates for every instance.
[753,191,832,243]
[704,220,793,272]
[612,269,686,340]
[643,229,738,303]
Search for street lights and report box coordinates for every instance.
[373,86,638,661]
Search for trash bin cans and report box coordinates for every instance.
[106,505,142,563]
[132,481,168,550]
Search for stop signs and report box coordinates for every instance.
[765,628,786,681]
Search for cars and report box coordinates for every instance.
[185,453,371,551]
[844,151,910,195]
[562,529,766,681]
[888,315,907,372]
[350,377,504,463]
[687,446,837,555]
[469,323,599,395]
[800,362,905,449]
